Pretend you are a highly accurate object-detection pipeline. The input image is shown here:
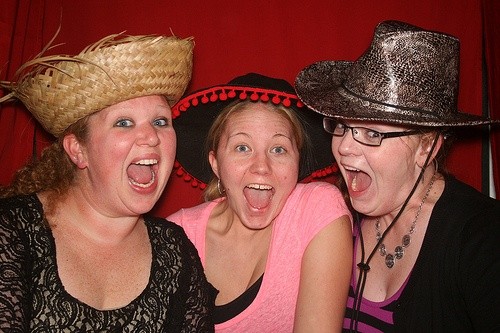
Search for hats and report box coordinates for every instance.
[294,20,500,127]
[0,21,195,138]
[171,72,339,191]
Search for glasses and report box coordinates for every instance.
[322,117,427,146]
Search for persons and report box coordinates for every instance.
[293,20,500,333]
[0,35,220,333]
[163,72,354,333]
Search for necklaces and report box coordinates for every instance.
[376,170,438,270]
